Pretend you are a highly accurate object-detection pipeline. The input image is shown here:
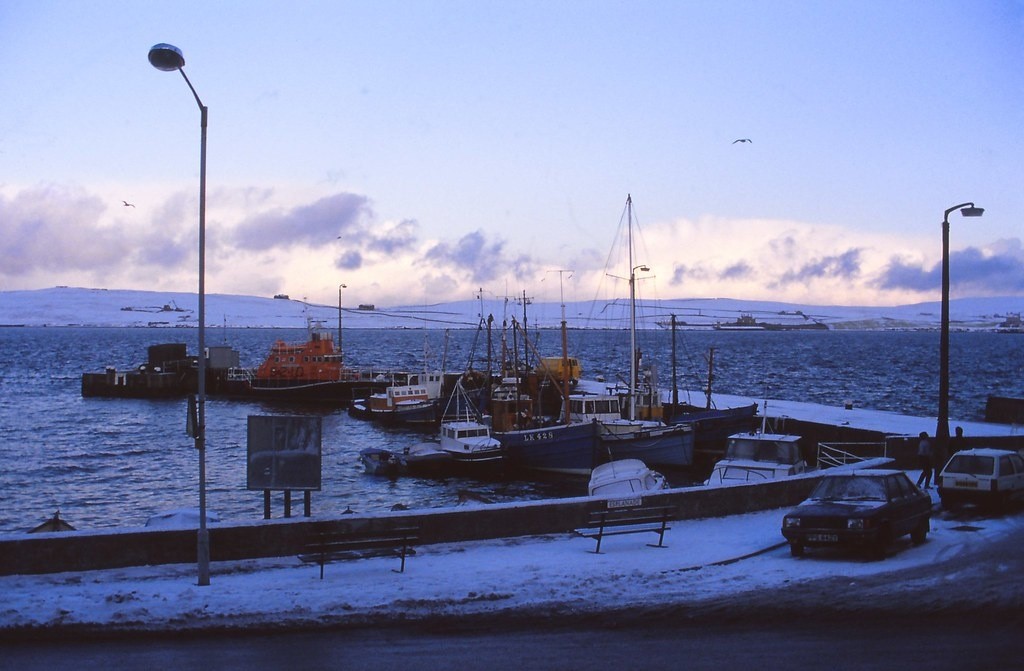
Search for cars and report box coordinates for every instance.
[936,447,1024,514]
[781,469,932,561]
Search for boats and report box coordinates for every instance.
[653,313,768,330]
[240,194,806,496]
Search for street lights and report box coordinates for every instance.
[338,284,348,367]
[148,42,211,585]
[933,202,984,485]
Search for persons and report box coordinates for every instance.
[916,432,933,489]
[948,427,970,458]
